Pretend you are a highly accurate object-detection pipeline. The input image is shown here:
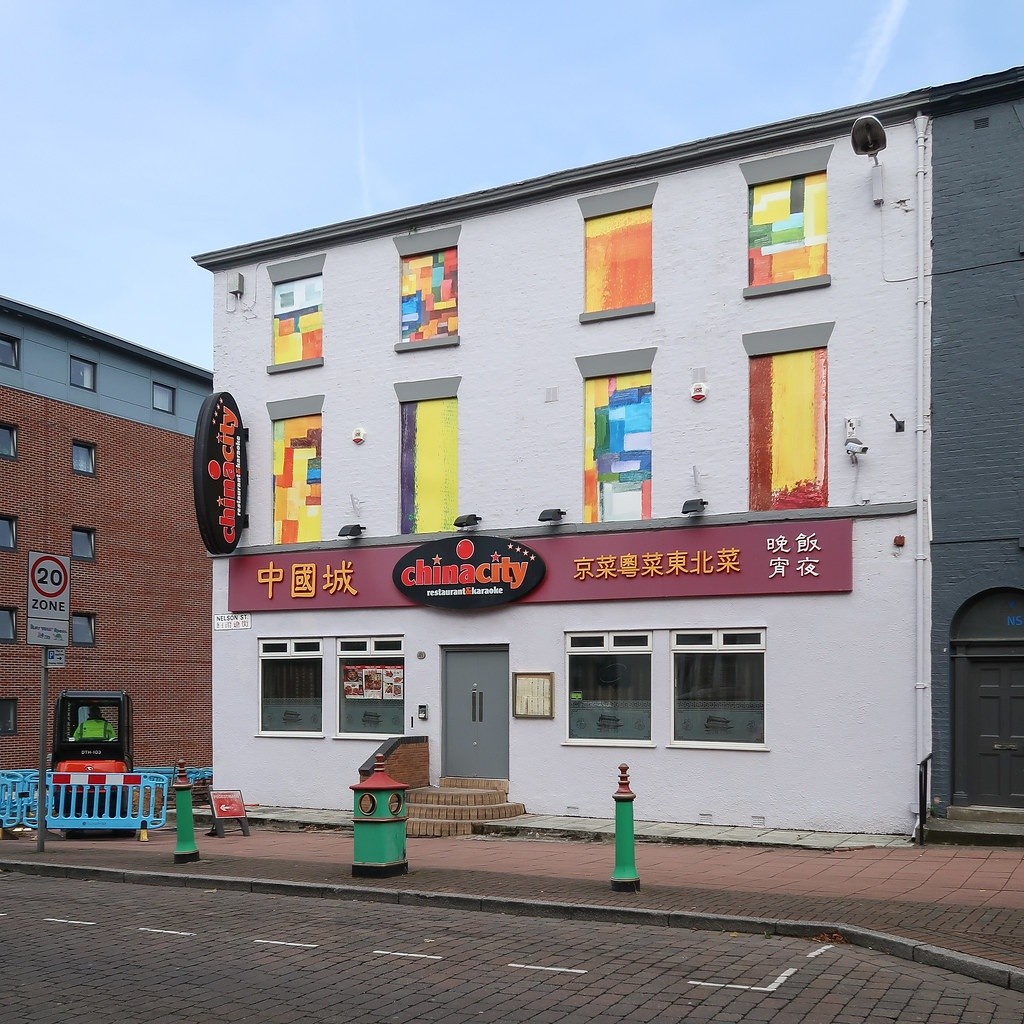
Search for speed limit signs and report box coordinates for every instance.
[27,551,68,598]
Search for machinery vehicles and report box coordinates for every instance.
[50,691,135,837]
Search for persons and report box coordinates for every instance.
[73,707,116,741]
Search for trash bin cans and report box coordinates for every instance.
[345,754,413,876]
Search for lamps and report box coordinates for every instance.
[852,114,888,167]
[538,509,565,523]
[453,514,482,527]
[682,499,708,517]
[338,524,366,537]
